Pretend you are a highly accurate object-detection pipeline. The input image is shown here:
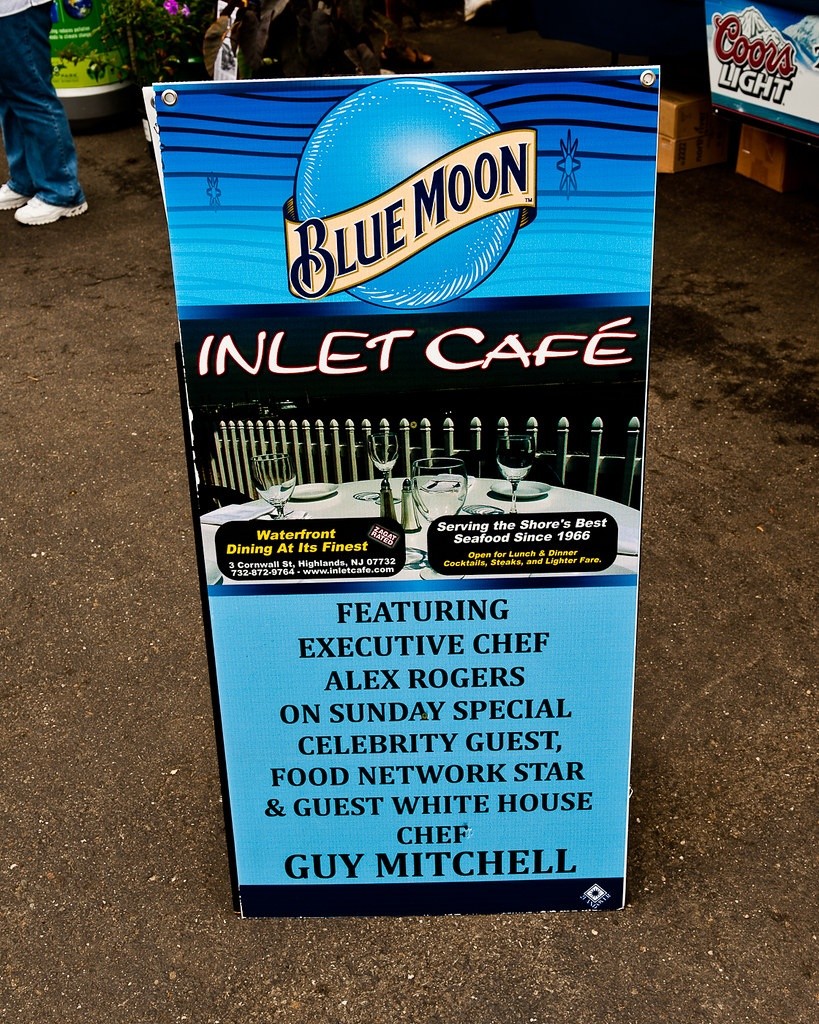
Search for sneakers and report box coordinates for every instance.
[0,183,88,226]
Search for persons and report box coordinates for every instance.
[0,0,90,227]
